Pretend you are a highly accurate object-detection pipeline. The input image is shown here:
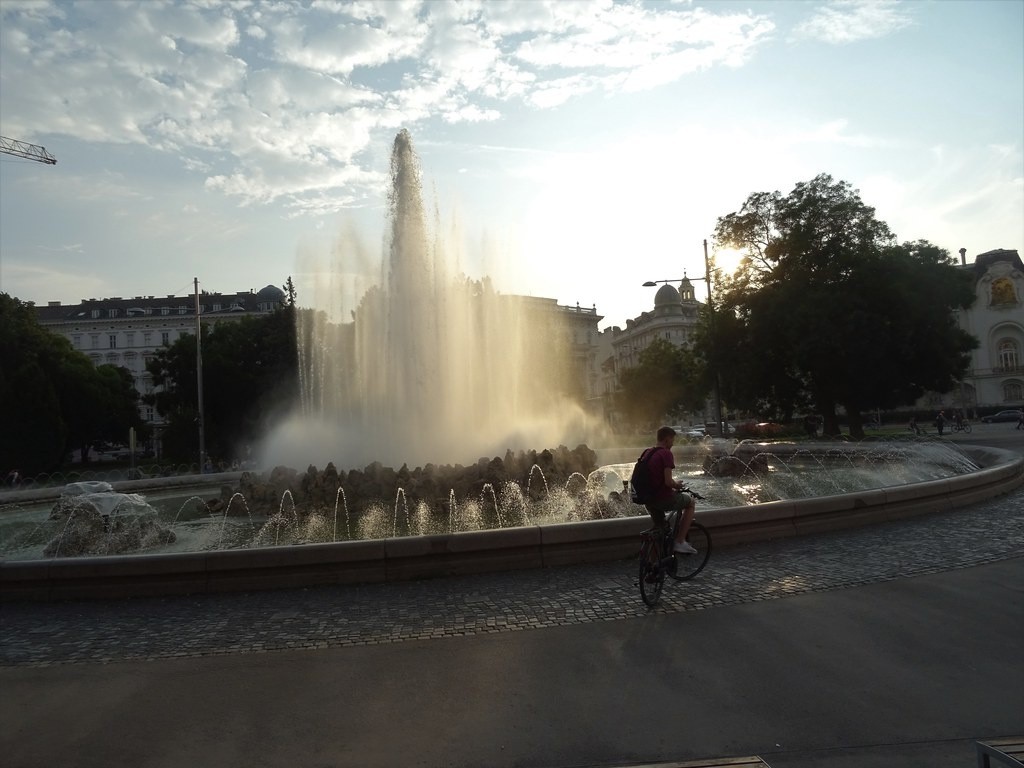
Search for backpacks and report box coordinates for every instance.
[631,447,664,505]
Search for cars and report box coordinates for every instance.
[980,409,1023,423]
[678,426,704,441]
[692,424,706,435]
[670,425,683,434]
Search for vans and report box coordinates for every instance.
[704,421,736,436]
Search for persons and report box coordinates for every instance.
[630,426,699,555]
[807,411,818,440]
[1015,408,1024,429]
[936,411,948,435]
[956,407,965,431]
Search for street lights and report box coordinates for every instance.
[641,239,725,440]
[192,278,244,475]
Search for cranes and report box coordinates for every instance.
[0,136,59,167]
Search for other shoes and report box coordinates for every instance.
[651,554,671,568]
[672,540,698,553]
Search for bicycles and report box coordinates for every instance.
[635,484,713,610]
[951,419,972,434]
